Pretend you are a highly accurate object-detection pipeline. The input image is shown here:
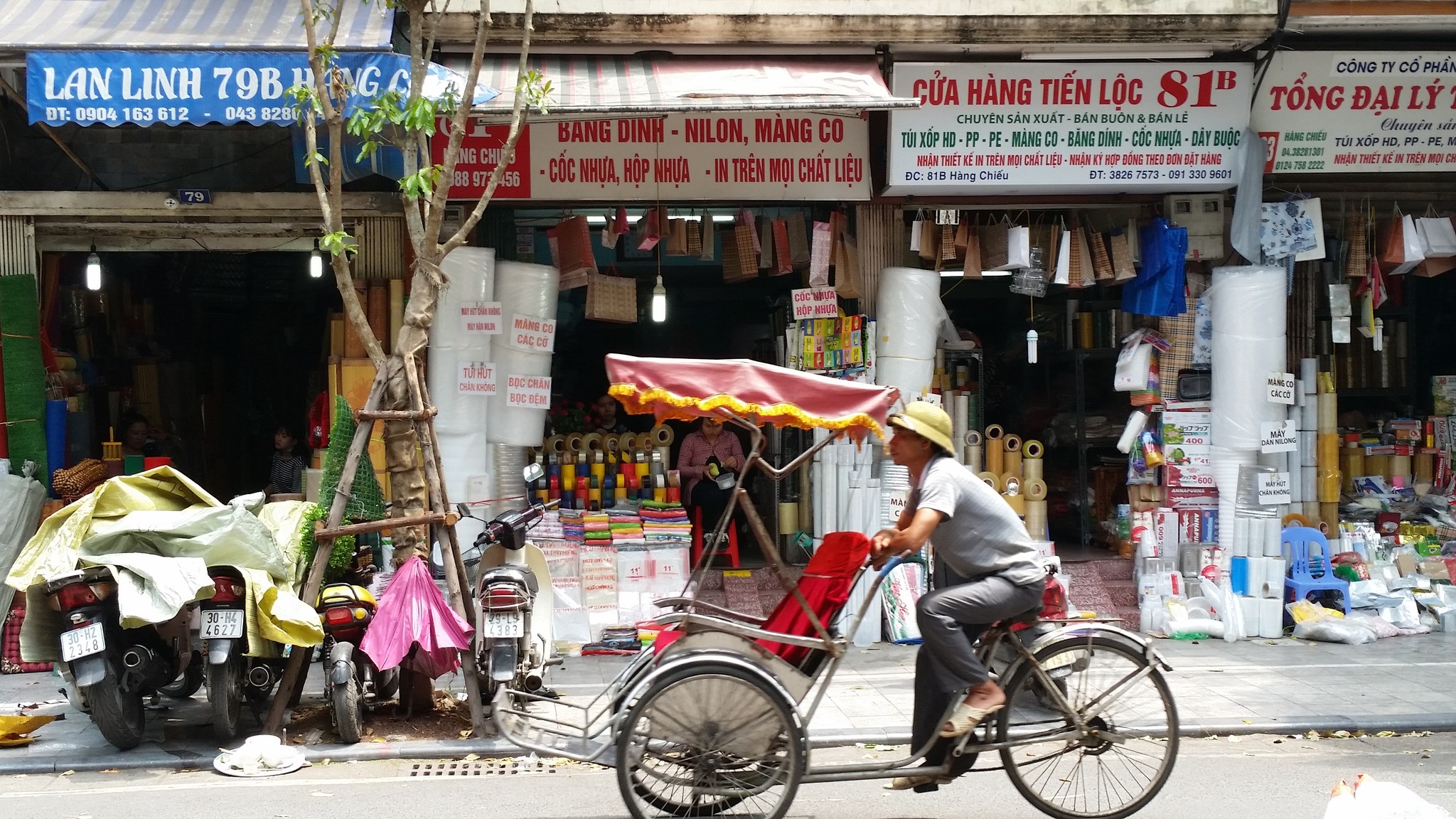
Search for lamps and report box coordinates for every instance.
[569,212,736,224]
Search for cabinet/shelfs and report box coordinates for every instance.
[1048,348,1128,551]
[1130,412,1224,598]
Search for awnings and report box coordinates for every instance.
[0,0,922,124]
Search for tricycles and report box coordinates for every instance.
[492,353,1180,819]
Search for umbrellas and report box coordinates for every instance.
[360,557,476,722]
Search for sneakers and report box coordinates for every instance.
[703,532,718,551]
[717,532,730,551]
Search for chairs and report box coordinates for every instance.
[655,531,872,672]
[1281,527,1352,615]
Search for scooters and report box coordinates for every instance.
[974,483,1095,710]
[315,516,398,744]
[455,463,563,700]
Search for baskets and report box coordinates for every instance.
[461,541,496,586]
[357,545,373,572]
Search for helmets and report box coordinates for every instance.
[886,401,955,457]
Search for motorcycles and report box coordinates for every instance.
[43,565,290,753]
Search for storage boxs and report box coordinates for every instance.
[1375,510,1442,557]
[1344,375,1456,495]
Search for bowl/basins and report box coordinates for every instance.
[1186,596,1217,620]
[716,472,736,490]
[1187,606,1210,620]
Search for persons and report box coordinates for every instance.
[868,400,1046,790]
[583,388,631,434]
[677,417,748,551]
[117,412,158,458]
[263,419,311,503]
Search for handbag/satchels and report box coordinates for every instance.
[766,206,793,276]
[721,214,762,280]
[601,206,630,249]
[809,204,866,299]
[650,205,670,239]
[585,261,637,324]
[783,201,811,267]
[547,211,598,291]
[1337,193,1456,338]
[687,207,702,256]
[1115,341,1161,407]
[755,206,773,268]
[1260,190,1326,261]
[699,207,714,261]
[910,206,1142,289]
[637,209,659,251]
[664,208,688,256]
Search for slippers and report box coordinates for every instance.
[882,767,952,790]
[938,700,1005,738]
